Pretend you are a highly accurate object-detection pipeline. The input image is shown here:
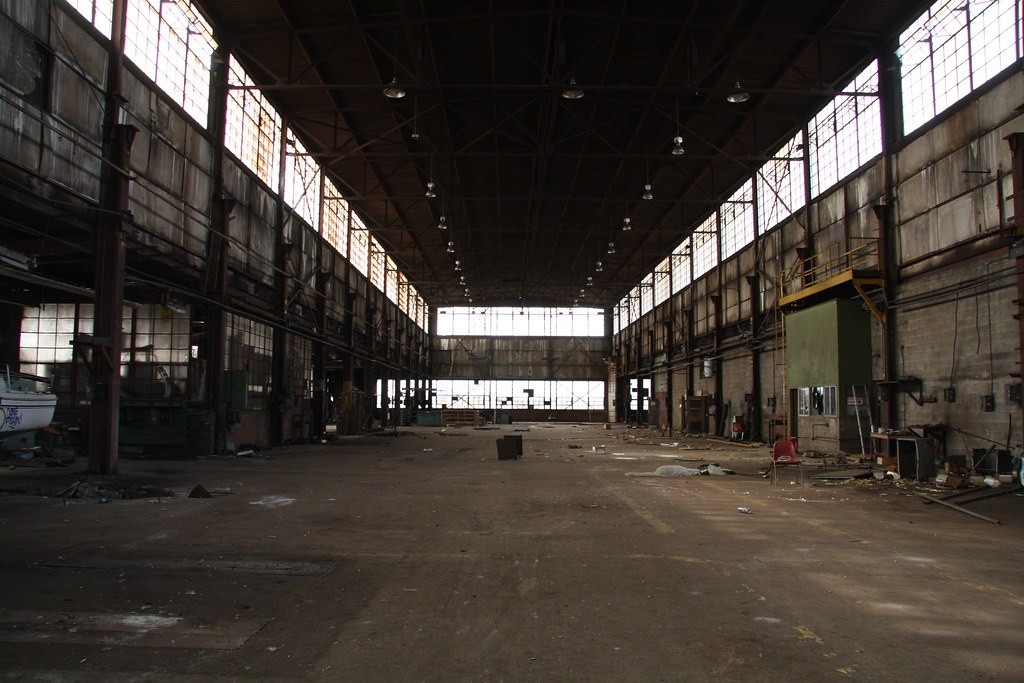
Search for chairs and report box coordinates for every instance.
[768,437,805,487]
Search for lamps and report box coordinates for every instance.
[562,76,584,98]
[672,137,684,154]
[383,76,406,98]
[642,185,653,200]
[447,242,455,253]
[726,80,749,103]
[607,242,615,254]
[437,217,447,230]
[623,217,632,231]
[425,182,437,197]
[573,262,603,307]
[455,260,471,296]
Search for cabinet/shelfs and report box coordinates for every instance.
[871,434,936,483]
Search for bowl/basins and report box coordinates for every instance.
[878,428,886,434]
